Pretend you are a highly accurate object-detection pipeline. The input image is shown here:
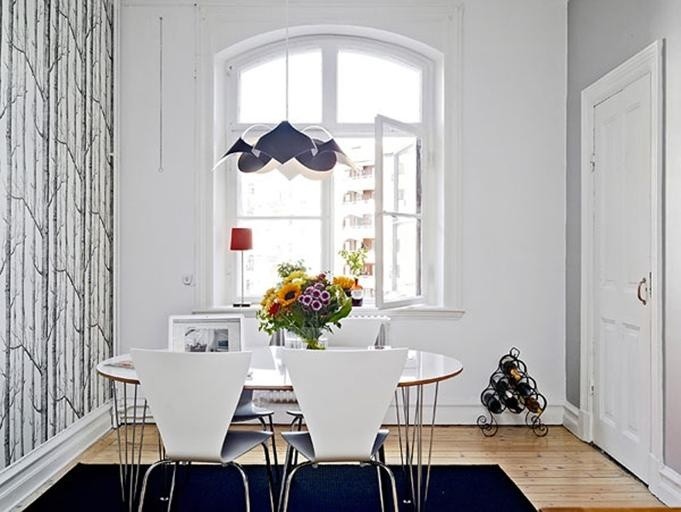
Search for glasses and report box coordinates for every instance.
[230,227,253,306]
[211,1,355,181]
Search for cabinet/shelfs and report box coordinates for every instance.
[484,357,543,416]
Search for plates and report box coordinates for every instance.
[477,348,549,437]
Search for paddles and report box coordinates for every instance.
[17,463,538,512]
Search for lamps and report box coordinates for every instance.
[277,348,409,512]
[130,348,276,512]
[286,316,384,477]
[231,317,279,473]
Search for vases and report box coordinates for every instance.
[255,237,373,349]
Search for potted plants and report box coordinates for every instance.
[167,314,245,352]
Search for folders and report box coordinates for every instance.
[254,316,390,402]
